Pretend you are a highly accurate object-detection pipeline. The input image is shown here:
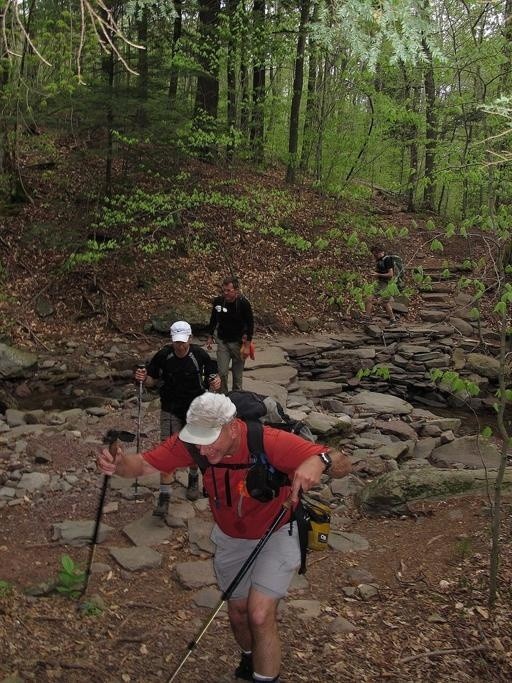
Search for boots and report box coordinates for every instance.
[185,472,199,500]
[151,492,170,516]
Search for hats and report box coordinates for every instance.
[178,390,236,446]
[169,319,191,342]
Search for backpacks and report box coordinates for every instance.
[382,254,404,278]
[224,389,317,490]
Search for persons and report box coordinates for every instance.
[95,390,353,682]
[136,318,222,516]
[203,276,252,392]
[363,242,400,329]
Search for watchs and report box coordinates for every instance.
[317,448,334,472]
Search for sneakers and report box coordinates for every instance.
[235,651,253,679]
[385,318,397,328]
